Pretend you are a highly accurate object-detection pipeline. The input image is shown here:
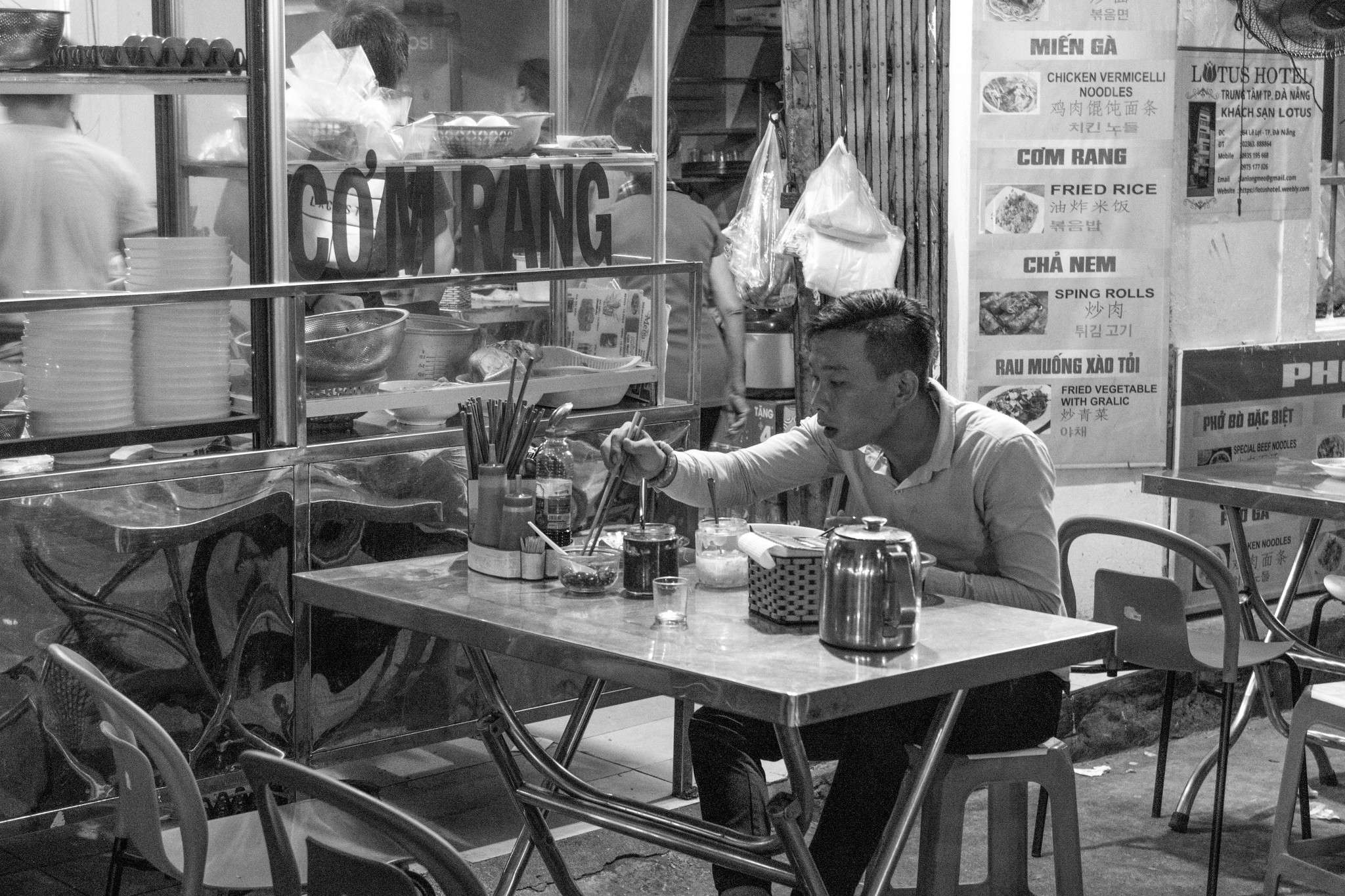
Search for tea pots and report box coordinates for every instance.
[818,515,922,650]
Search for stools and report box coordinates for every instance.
[1265,680,1345,896]
[866,737,1083,896]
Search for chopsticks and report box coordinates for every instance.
[458,357,546,480]
[580,411,645,556]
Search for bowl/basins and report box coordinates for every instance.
[378,379,461,426]
[921,551,937,582]
[747,522,827,538]
[428,109,555,156]
[0,235,233,465]
[554,544,622,593]
[386,313,480,380]
[981,72,1038,112]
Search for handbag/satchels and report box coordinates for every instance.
[804,135,889,245]
[720,109,797,311]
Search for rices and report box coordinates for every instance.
[994,190,1038,234]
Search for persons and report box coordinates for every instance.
[599,287,1072,895]
[503,58,576,154]
[0,92,159,301]
[570,97,749,456]
[210,1,457,345]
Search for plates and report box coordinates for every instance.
[1209,451,1230,465]
[985,186,1045,234]
[1314,533,1345,576]
[1312,457,1344,479]
[1195,547,1227,588]
[984,0,1045,21]
[978,384,1051,432]
[533,141,632,155]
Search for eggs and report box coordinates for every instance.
[122,35,234,66]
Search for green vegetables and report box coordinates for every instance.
[987,386,1049,425]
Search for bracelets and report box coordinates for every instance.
[643,440,677,488]
[309,294,323,313]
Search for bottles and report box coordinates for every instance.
[694,517,749,590]
[473,428,574,548]
[623,522,680,596]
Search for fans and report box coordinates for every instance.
[1224,0,1345,61]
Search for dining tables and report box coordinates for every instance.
[1141,458,1345,833]
[294,521,1118,896]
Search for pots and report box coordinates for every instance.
[285,169,412,272]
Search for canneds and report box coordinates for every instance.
[695,517,750,588]
[622,522,679,598]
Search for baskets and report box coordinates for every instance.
[420,125,522,158]
[233,116,349,159]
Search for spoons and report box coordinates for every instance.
[527,521,597,574]
[547,402,574,429]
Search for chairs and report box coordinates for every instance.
[1030,517,1345,896]
[37,643,489,896]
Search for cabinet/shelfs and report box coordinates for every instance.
[0,0,674,474]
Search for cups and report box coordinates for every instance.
[652,576,689,627]
[520,551,546,580]
[513,252,550,303]
